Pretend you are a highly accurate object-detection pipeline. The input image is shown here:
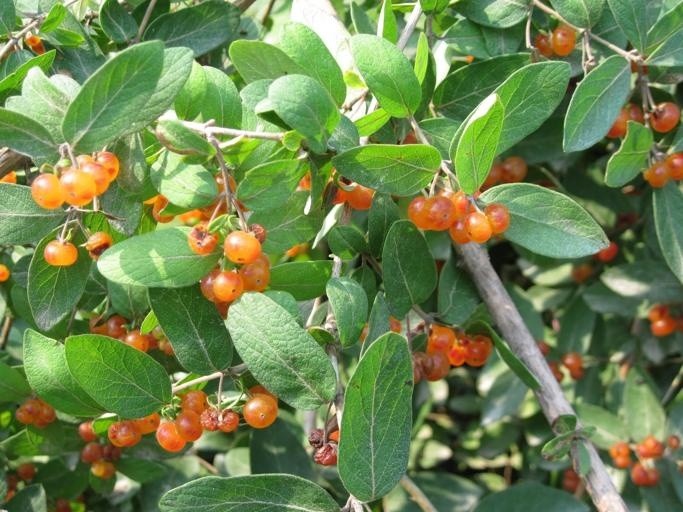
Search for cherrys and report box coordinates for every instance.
[0,24,683,511]
